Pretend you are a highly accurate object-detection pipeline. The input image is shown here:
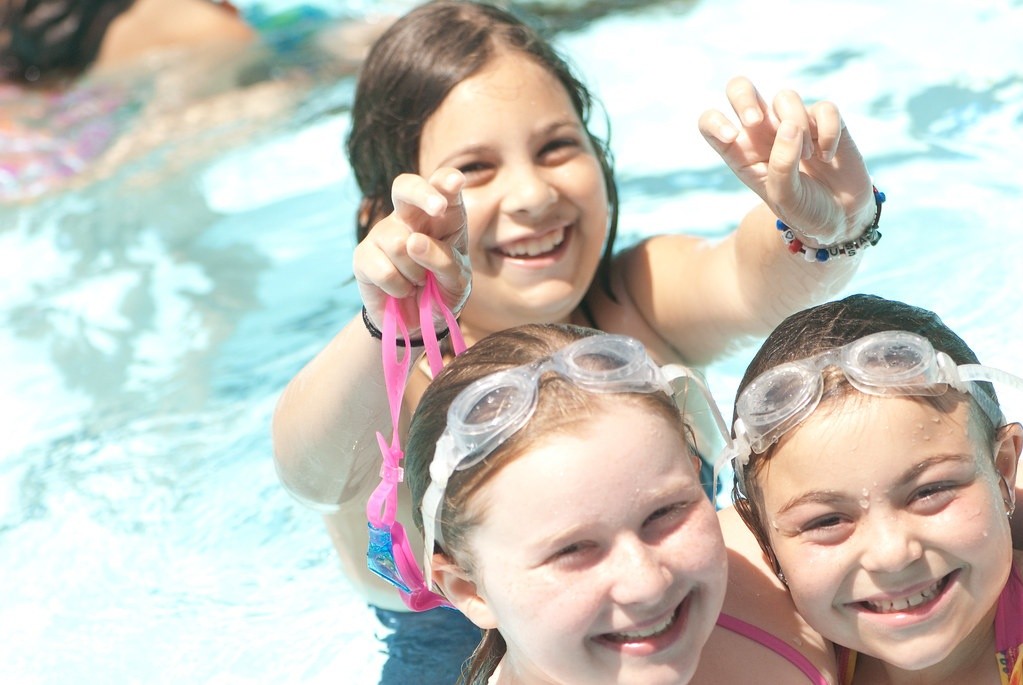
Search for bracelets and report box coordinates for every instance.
[359,302,462,346]
[775,186,886,263]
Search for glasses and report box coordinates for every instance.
[366,269,474,610]
[420,334,735,596]
[711,331,1007,508]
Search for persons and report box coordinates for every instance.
[403,324,1023,685]
[272,0,886,613]
[0,0,694,234]
[730,294,1023,684]
